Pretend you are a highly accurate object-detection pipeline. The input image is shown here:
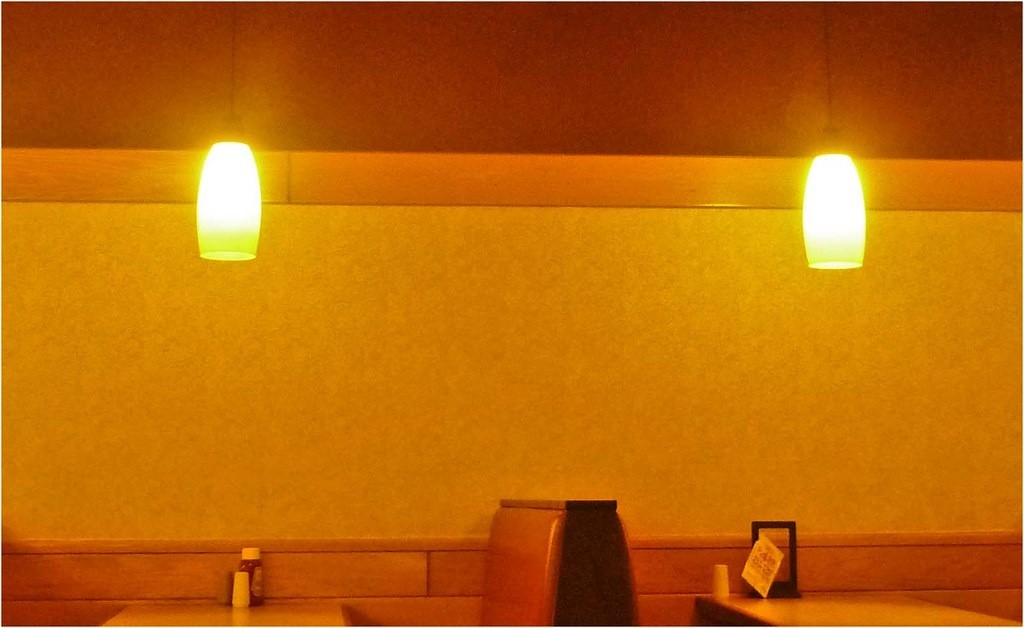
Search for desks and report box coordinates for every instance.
[692,592,1022,627]
[101,604,351,626]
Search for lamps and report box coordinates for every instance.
[197,2,262,265]
[801,2,866,269]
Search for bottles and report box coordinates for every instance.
[239,547,264,606]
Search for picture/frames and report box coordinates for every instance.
[751,521,801,598]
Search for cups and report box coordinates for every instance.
[712,564,729,597]
[232,571,251,607]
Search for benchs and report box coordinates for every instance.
[477,499,640,626]
